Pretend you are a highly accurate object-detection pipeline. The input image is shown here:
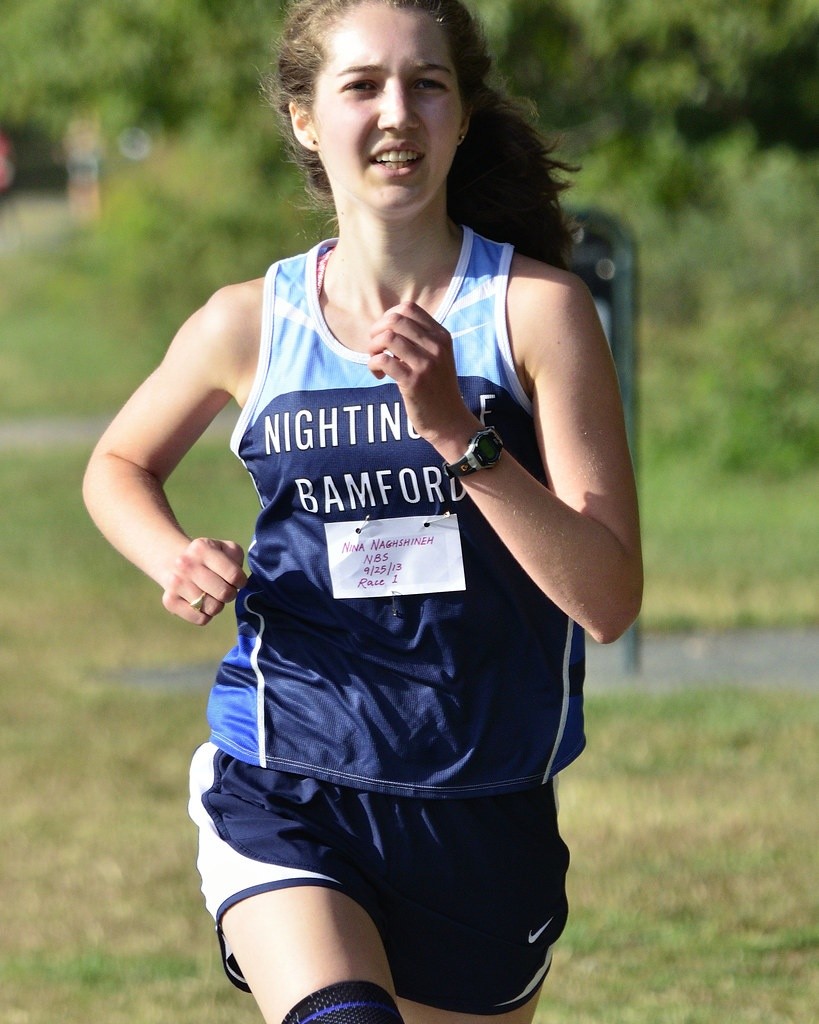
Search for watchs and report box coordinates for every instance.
[441,426,505,478]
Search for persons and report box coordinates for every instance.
[83,0,646,1022]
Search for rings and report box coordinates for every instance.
[188,592,207,611]
